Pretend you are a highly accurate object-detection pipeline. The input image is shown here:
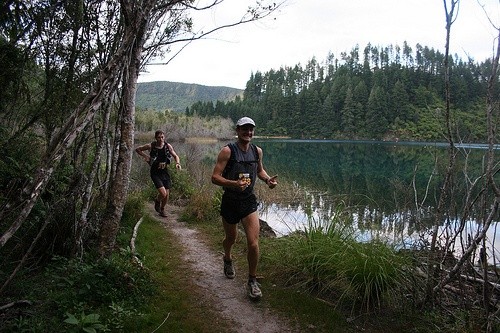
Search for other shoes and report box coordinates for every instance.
[155,199,161,212]
[159,208,168,217]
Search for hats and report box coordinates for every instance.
[236,117,255,127]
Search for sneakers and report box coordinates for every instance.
[248,281,262,297]
[224,261,235,278]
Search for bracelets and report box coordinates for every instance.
[176,162,180,165]
[265,177,269,184]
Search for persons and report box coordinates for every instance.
[135,130,181,218]
[210,116,279,301]
